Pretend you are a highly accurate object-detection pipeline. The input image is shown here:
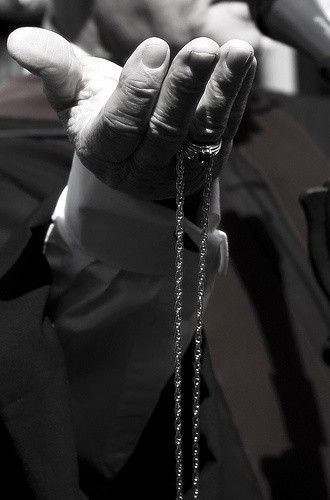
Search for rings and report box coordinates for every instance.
[182,137,226,159]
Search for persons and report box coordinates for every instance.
[1,0,329,500]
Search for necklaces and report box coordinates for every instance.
[176,143,214,500]
